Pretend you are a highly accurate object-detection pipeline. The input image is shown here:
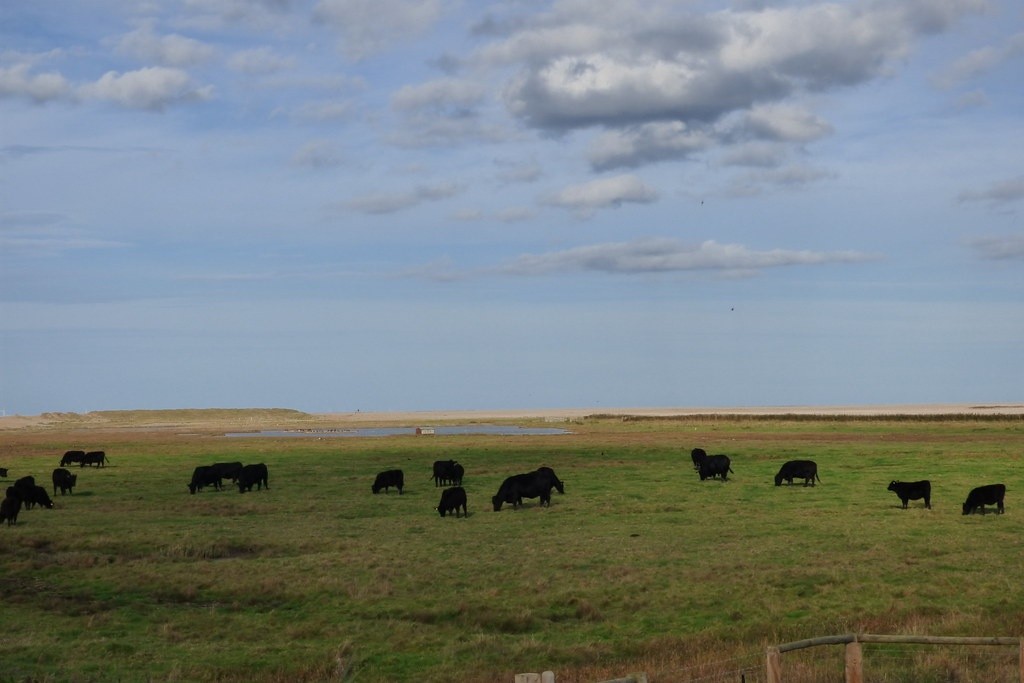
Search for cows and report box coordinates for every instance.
[186,461,269,495]
[429,458,468,519]
[491,466,565,512]
[886,479,931,510]
[690,448,735,483]
[371,469,404,496]
[774,459,821,488]
[961,483,1006,516]
[0,450,110,527]
